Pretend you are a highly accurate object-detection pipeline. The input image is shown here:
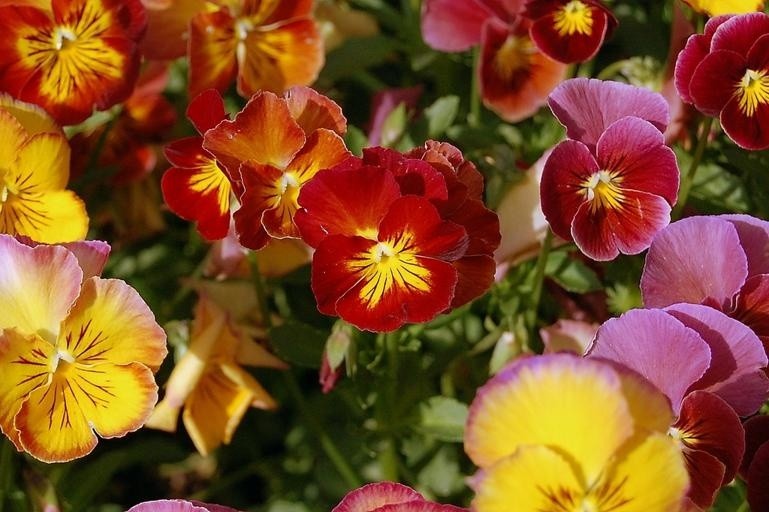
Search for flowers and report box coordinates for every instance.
[1,0,769,511]
